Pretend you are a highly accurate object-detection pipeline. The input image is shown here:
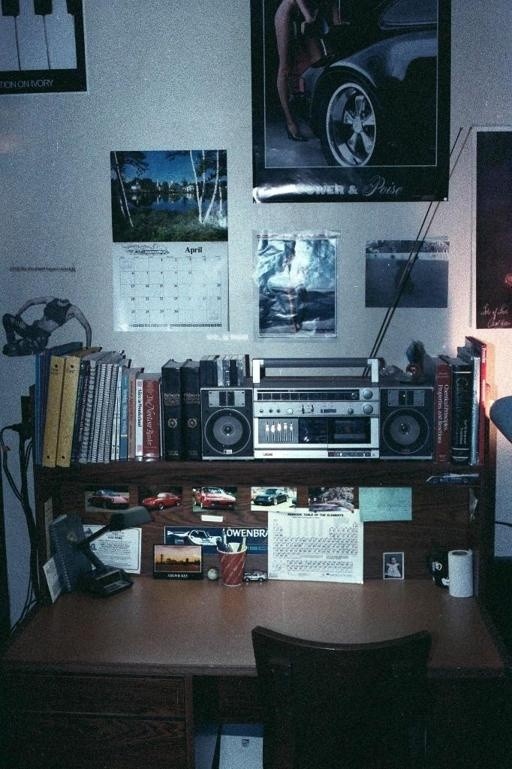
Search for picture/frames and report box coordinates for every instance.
[382,552,405,581]
[469,125,512,332]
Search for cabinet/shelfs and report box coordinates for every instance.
[4,676,193,768]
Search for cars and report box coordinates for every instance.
[83,480,290,550]
[295,1,439,169]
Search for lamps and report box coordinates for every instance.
[77,505,154,600]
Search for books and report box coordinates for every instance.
[430,335,488,466]
[49,509,93,593]
[34,340,203,469]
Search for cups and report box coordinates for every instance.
[217,543,247,588]
[430,551,448,587]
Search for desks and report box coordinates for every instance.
[0,571,509,769]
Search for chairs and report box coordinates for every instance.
[251,625,434,767]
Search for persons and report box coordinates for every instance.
[384,556,401,578]
[0,295,91,356]
[272,0,325,143]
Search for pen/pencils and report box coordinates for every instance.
[216,529,243,552]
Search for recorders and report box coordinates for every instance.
[200,358,435,459]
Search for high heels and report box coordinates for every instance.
[286,123,308,141]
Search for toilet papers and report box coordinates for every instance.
[446,545,476,598]
